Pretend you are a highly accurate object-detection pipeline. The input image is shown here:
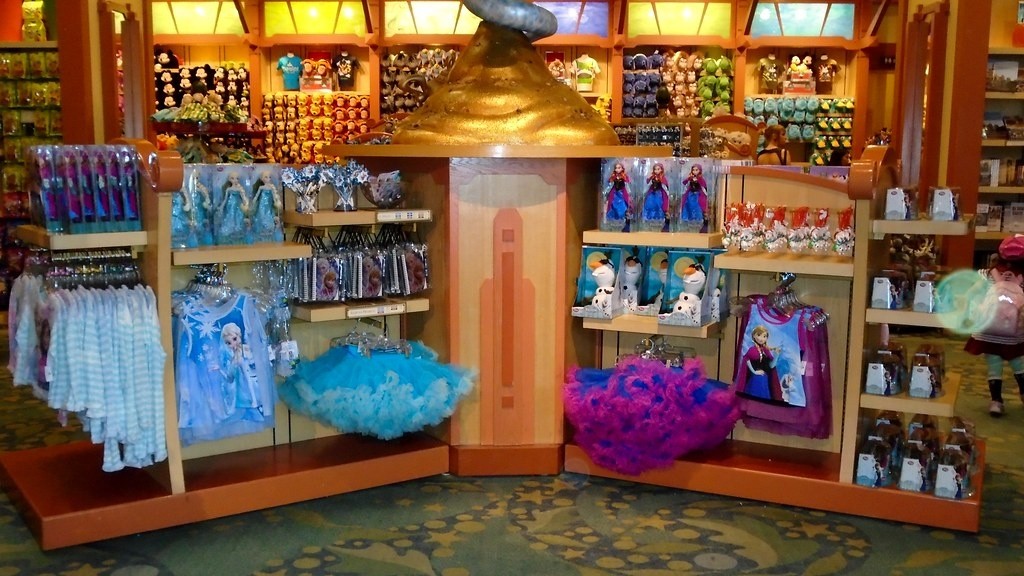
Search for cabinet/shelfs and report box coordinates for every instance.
[1,139,985,552]
[153,35,871,151]
[974,48,1024,239]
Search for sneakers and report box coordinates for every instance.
[1021,395,1024,404]
[989,400,1004,415]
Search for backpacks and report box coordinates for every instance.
[978,267,1024,338]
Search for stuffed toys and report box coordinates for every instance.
[614,49,750,161]
[743,49,854,166]
[261,57,369,166]
[149,44,249,119]
[589,246,722,326]
[370,46,460,146]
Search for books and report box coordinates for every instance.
[974,57,1024,233]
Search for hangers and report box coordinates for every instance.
[19,240,156,294]
[728,273,830,332]
[170,263,258,316]
[615,332,698,373]
[330,317,415,358]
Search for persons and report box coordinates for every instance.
[252,170,282,243]
[217,171,249,243]
[754,125,792,167]
[185,169,214,248]
[604,163,633,222]
[642,162,672,221]
[169,179,191,244]
[960,232,1024,417]
[680,163,708,222]
[828,148,853,168]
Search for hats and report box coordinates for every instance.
[998,233,1024,260]
